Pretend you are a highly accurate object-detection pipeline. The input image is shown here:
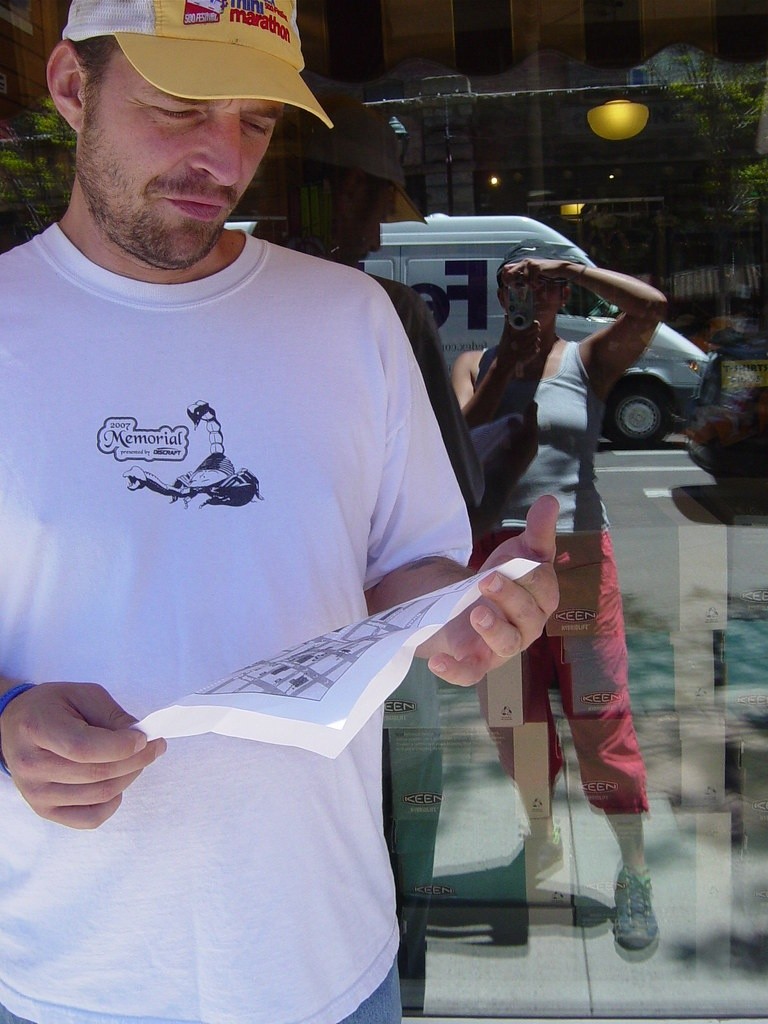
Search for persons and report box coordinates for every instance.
[451,238,668,959]
[0,0,560,1023]
[252,74,491,1015]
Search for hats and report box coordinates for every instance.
[495,239,568,288]
[62,0,335,130]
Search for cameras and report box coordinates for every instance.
[508,283,534,330]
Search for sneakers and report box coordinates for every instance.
[614,867,658,950]
[511,827,563,892]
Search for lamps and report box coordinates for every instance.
[588,98,649,142]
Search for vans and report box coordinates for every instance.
[224,214,719,451]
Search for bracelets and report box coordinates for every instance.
[0,683,37,776]
